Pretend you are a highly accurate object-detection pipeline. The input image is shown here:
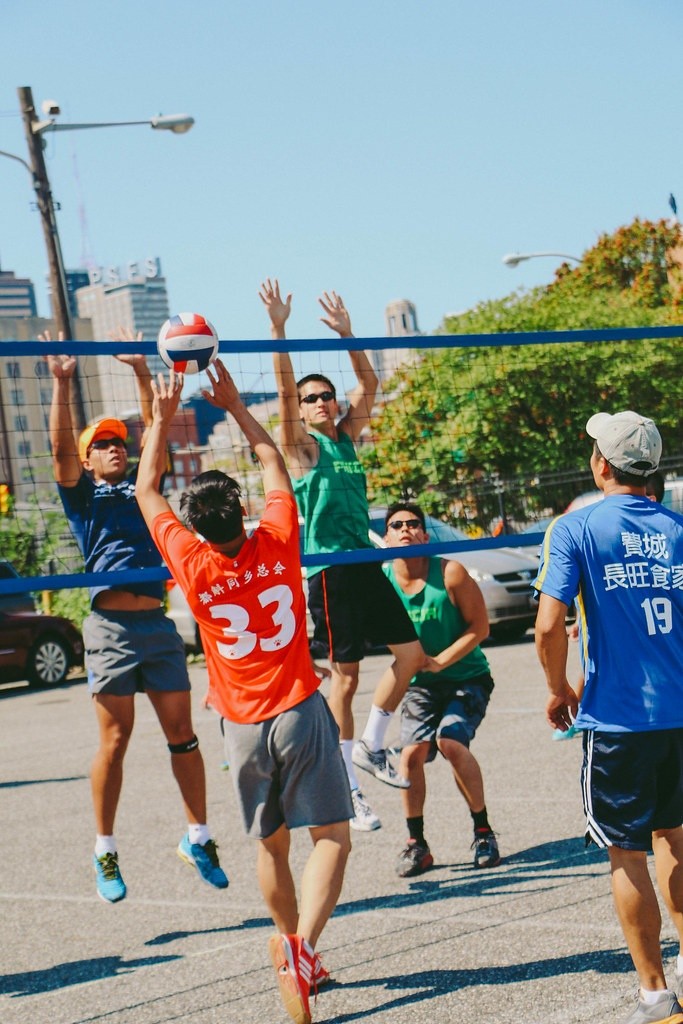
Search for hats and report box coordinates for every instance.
[79,418,128,461]
[587,410,663,477]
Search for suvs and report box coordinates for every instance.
[160,505,546,656]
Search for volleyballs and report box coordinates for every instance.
[157,310,221,375]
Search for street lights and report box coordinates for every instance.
[16,85,194,437]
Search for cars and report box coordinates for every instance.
[0,558,86,690]
[560,476,683,539]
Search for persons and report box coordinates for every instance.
[379,503,500,876]
[530,409,683,1024]
[37,325,230,902]
[135,355,352,1022]
[259,279,409,830]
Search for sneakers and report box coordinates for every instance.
[396,839,432,878]
[348,789,381,833]
[662,970,683,1008]
[176,833,231,888]
[470,827,501,869]
[93,850,127,903]
[622,989,683,1024]
[351,740,412,788]
[270,934,322,1024]
[313,967,331,985]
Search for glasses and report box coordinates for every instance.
[387,519,421,529]
[300,391,335,404]
[88,436,123,453]
